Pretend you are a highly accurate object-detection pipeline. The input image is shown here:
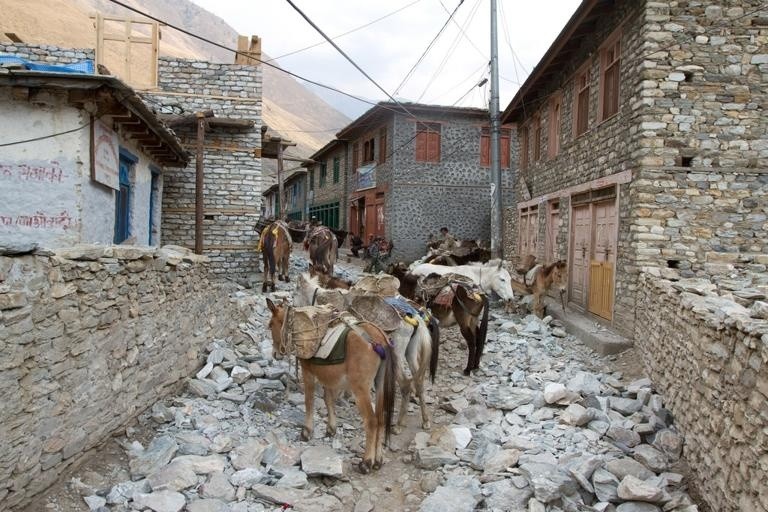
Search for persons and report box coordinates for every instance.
[348,226,457,275]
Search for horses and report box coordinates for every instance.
[304,223,338,277]
[264,263,440,475]
[261,222,291,294]
[406,257,515,304]
[385,262,491,377]
[503,259,568,317]
